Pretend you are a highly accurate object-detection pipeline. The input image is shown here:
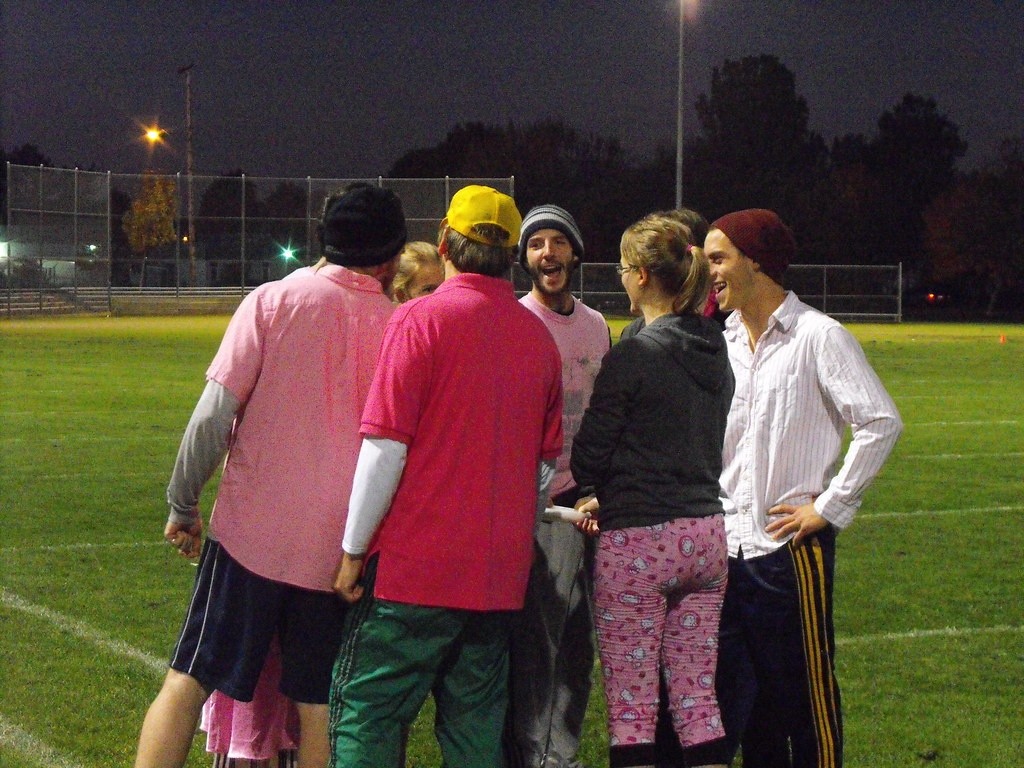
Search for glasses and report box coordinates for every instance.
[616,265,638,275]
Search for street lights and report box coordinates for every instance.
[139,129,166,287]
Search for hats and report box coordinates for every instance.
[318,181,408,267]
[712,209,796,285]
[440,185,522,247]
[517,205,585,275]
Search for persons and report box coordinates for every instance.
[196,185,736,768]
[134,179,405,767]
[703,209,902,768]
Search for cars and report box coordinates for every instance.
[883,268,1024,321]
